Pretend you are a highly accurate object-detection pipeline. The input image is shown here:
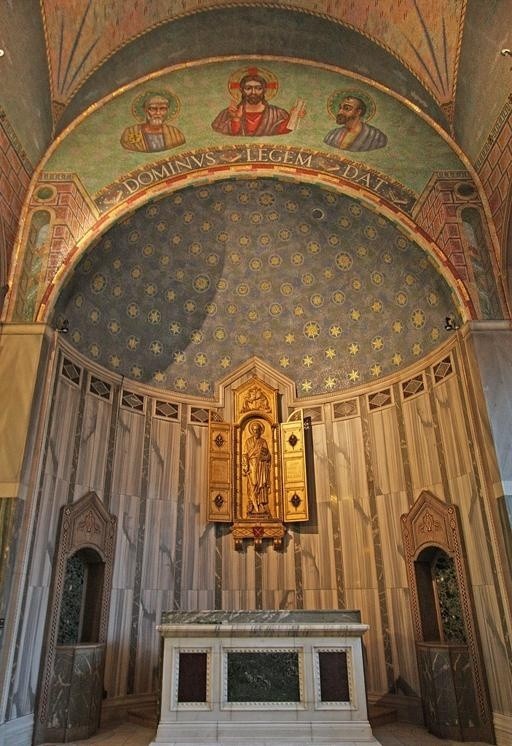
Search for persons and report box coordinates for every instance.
[241,423,271,514]
[323,95,388,152]
[211,75,306,136]
[121,96,186,153]
